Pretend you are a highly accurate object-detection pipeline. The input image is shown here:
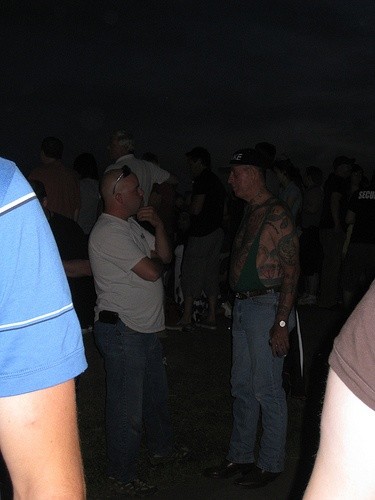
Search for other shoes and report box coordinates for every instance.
[193,311,217,330]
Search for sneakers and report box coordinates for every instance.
[106,474,158,496]
[166,440,193,463]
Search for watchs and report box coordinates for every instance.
[274,319,288,327]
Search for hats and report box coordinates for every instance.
[230,148,270,169]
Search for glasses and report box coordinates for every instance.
[113,164,131,194]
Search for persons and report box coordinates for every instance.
[301,276,375,500]
[88,165,186,496]
[255,155,375,310]
[0,156,89,500]
[25,180,96,329]
[166,146,227,330]
[142,152,247,231]
[205,147,301,488]
[29,136,82,220]
[104,129,178,206]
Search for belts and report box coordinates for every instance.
[206,459,254,478]
[234,285,280,299]
[234,471,280,487]
